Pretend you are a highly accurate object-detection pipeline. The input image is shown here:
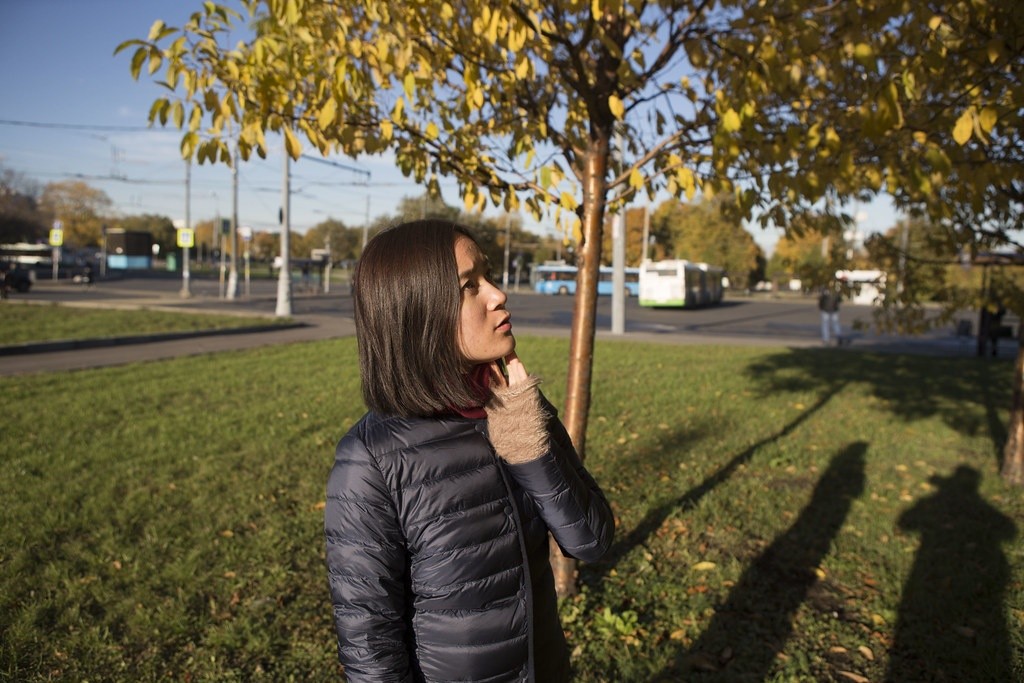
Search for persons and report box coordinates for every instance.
[977,294,1006,358]
[323,219,615,683]
[819,282,843,342]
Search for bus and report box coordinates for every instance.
[529,259,639,296]
[638,258,724,309]
